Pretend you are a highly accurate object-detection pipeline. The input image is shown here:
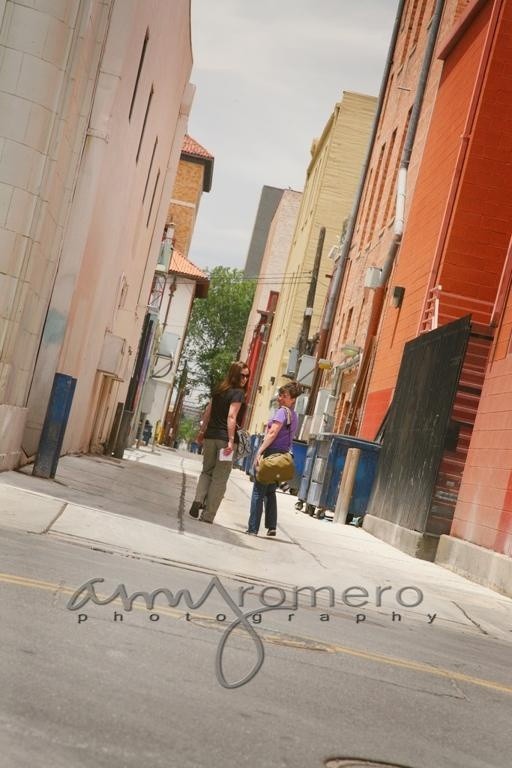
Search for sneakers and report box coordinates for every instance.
[245,530,256,536]
[266,529,275,536]
[189,500,202,518]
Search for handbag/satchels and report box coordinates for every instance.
[256,452,294,485]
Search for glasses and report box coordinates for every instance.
[240,373,249,378]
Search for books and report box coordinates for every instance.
[219,448,234,461]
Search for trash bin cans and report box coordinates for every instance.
[190,442,198,454]
[295,432,384,528]
[242,434,310,496]
[135,424,153,446]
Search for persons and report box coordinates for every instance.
[187,361,251,525]
[240,382,303,537]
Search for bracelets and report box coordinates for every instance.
[197,429,205,435]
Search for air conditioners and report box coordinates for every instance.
[364,266,382,288]
[156,332,181,359]
